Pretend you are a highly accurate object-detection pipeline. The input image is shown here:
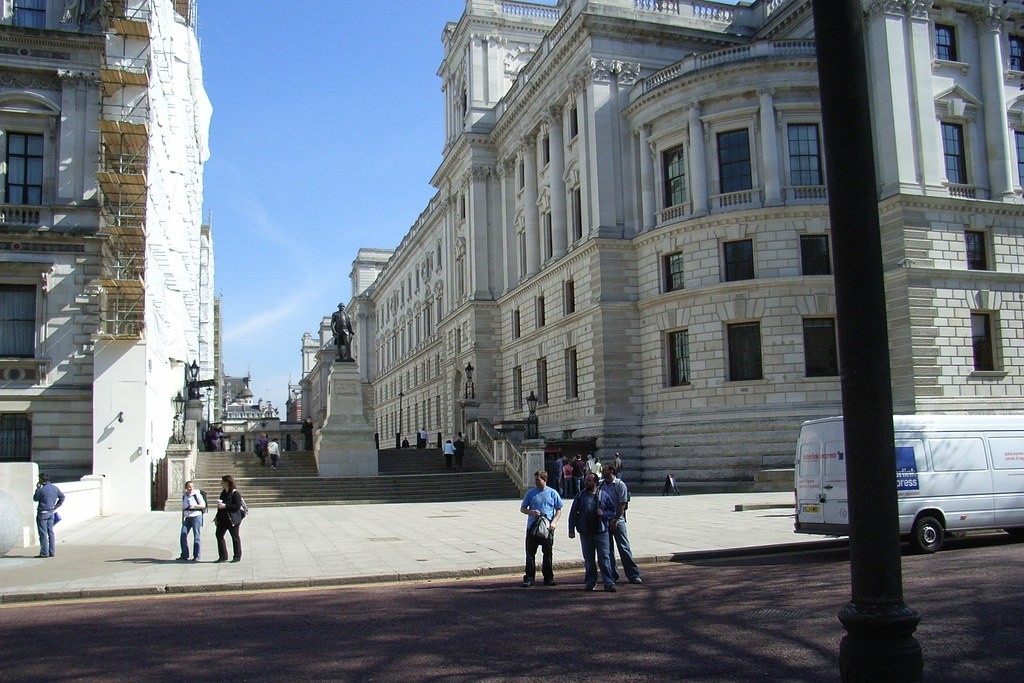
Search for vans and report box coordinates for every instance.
[793,413,1024,554]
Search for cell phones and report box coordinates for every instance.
[39,481,42,484]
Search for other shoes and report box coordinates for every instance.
[34,553,55,557]
[192,557,199,562]
[629,576,643,584]
[176,556,189,561]
[544,579,557,586]
[215,557,228,563]
[230,558,240,563]
[604,584,617,592]
[523,581,534,586]
[585,582,598,591]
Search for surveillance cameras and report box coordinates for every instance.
[472,341,475,345]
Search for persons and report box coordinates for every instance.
[402,437,410,450]
[201,426,225,452]
[596,463,642,584]
[331,303,355,360]
[545,450,624,499]
[213,475,248,563]
[521,470,563,587]
[569,472,618,593]
[33,473,65,558]
[254,433,269,466]
[300,416,314,450]
[267,438,280,470]
[442,436,466,470]
[176,481,206,561]
[418,426,429,449]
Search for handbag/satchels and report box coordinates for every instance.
[53,512,61,525]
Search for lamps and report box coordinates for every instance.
[118,412,124,422]
[188,360,200,400]
[465,362,475,399]
[525,390,540,439]
[173,391,187,444]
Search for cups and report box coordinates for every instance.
[218,500,223,503]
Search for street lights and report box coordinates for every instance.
[206,386,212,431]
[398,391,404,433]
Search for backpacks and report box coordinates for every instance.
[528,514,553,545]
[230,489,249,519]
[183,489,209,514]
